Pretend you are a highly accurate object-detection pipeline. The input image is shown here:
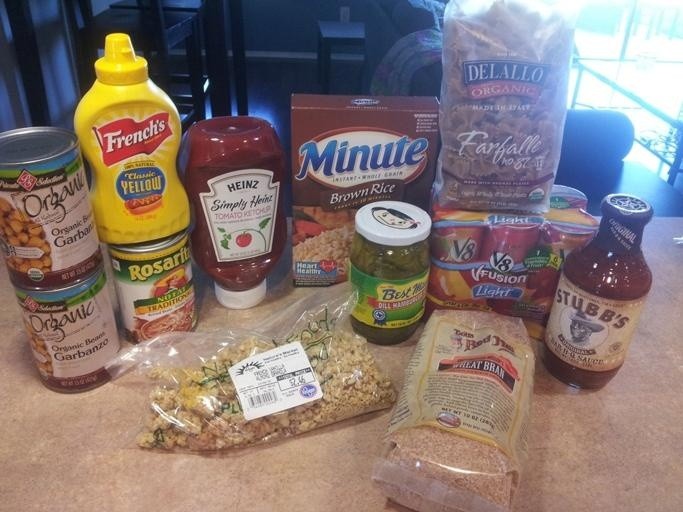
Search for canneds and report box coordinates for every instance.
[349,185,601,346]
[10,264,124,393]
[108,229,198,346]
[0,126,103,291]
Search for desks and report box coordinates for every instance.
[0,218,682,507]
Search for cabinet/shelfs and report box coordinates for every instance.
[74,0,245,137]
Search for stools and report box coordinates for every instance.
[317,22,366,99]
